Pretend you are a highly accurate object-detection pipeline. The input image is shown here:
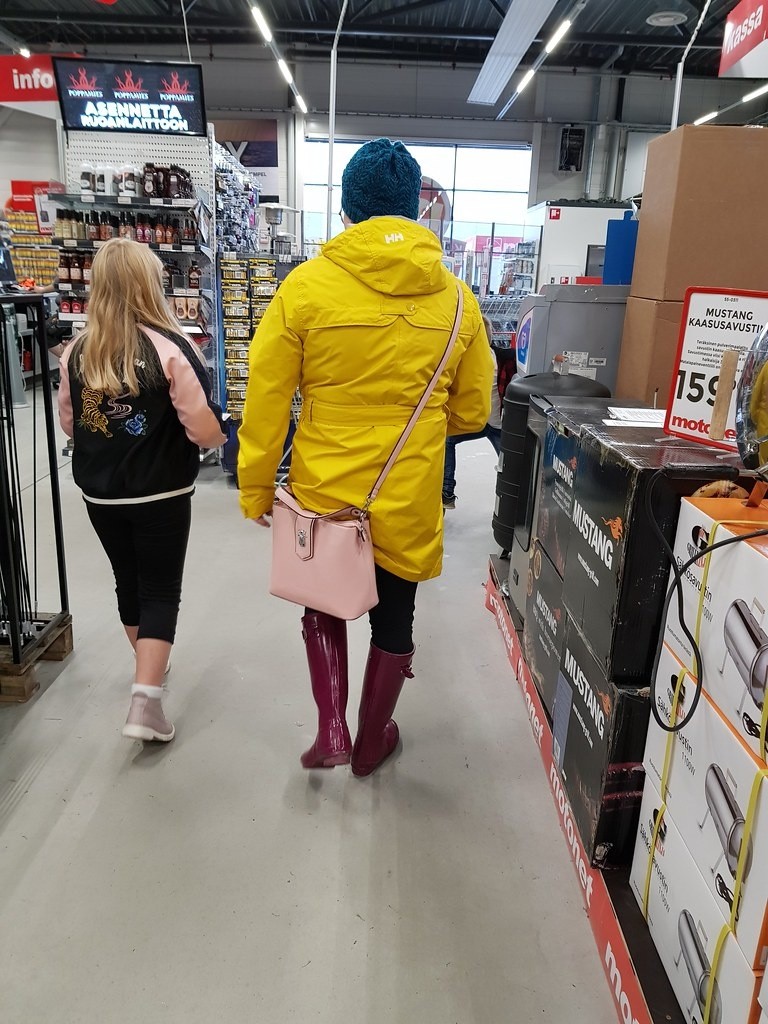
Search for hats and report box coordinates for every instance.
[341,137,422,223]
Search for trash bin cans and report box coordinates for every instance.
[492,371,611,561]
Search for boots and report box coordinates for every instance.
[351,639,415,778]
[299,610,354,770]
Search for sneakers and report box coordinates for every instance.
[122,683,177,744]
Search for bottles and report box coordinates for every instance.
[188,260,201,287]
[56,208,205,245]
[59,249,96,283]
[506,241,535,252]
[81,161,196,199]
[160,257,182,288]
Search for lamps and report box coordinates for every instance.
[246,0,309,115]
[494,0,768,127]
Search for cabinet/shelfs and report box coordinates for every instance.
[0,291,59,390]
[512,239,539,294]
[46,118,264,464]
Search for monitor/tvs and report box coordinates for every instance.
[51,56,208,138]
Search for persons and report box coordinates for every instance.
[237,138,489,776]
[29,273,70,390]
[58,238,231,742]
[442,314,502,509]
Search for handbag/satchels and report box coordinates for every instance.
[264,483,380,619]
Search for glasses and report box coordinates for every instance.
[338,207,345,223]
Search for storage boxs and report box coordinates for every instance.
[217,257,279,419]
[628,122,768,302]
[614,297,684,408]
[520,395,768,1024]
